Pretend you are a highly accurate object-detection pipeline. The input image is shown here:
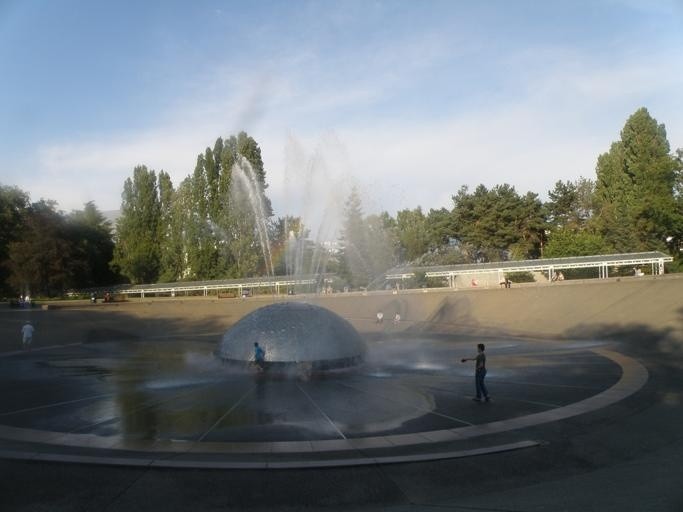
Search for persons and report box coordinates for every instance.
[316,285,333,296]
[21,321,35,351]
[391,311,401,329]
[461,344,491,401]
[557,271,564,282]
[254,341,266,371]
[375,310,383,326]
[551,271,558,284]
[19,295,30,308]
